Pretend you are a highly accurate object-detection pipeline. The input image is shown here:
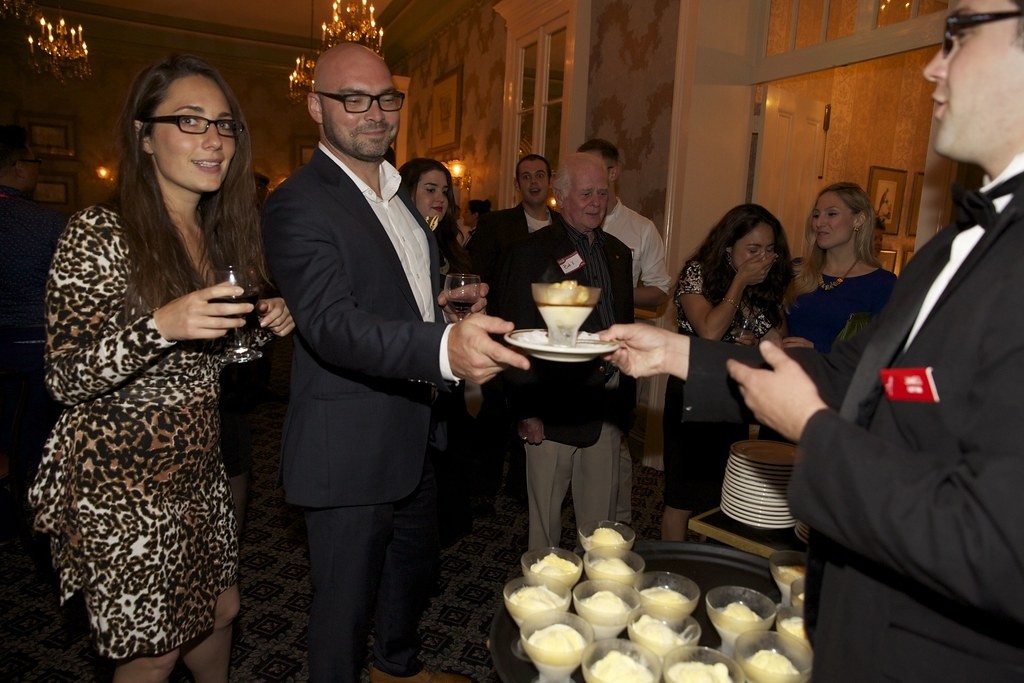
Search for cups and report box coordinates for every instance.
[533,284,601,347]
[502,519,806,683]
[736,315,755,337]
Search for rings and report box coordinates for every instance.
[522,436,528,440]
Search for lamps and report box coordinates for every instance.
[24,0,94,91]
[441,160,472,193]
[288,0,385,106]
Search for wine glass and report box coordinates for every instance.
[444,274,481,321]
[213,265,263,363]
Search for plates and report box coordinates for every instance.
[503,329,619,362]
[720,440,808,542]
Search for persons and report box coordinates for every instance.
[0,124,70,496]
[218,140,671,553]
[760,181,897,350]
[28,53,297,683]
[662,203,794,541]
[259,42,530,683]
[597,0,1024,683]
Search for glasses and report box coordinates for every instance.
[942,11,1024,57]
[314,91,405,113]
[12,158,42,166]
[145,115,245,138]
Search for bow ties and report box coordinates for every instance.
[950,181,997,234]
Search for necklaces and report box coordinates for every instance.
[816,258,858,291]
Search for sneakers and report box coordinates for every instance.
[368,660,472,683]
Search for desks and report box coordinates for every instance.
[688,506,807,559]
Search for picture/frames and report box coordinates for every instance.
[429,62,464,154]
[905,171,924,236]
[33,169,79,210]
[878,248,898,273]
[13,108,81,162]
[867,164,908,236]
[287,132,319,174]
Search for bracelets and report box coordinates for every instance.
[722,297,738,308]
